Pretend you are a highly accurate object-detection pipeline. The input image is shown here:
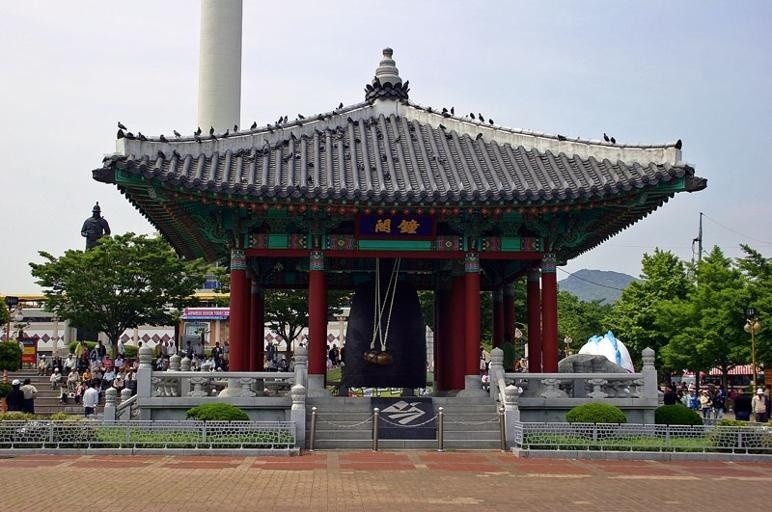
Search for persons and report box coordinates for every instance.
[5,378,38,416]
[38,340,287,417]
[756,365,764,375]
[515,357,528,373]
[298,343,305,349]
[326,343,344,369]
[480,346,490,391]
[658,381,770,422]
[81,201,110,249]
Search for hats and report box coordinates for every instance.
[756,388,763,394]
[12,379,19,386]
[41,344,134,379]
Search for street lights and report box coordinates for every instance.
[0,302,24,413]
[743,318,762,396]
[563,335,573,356]
